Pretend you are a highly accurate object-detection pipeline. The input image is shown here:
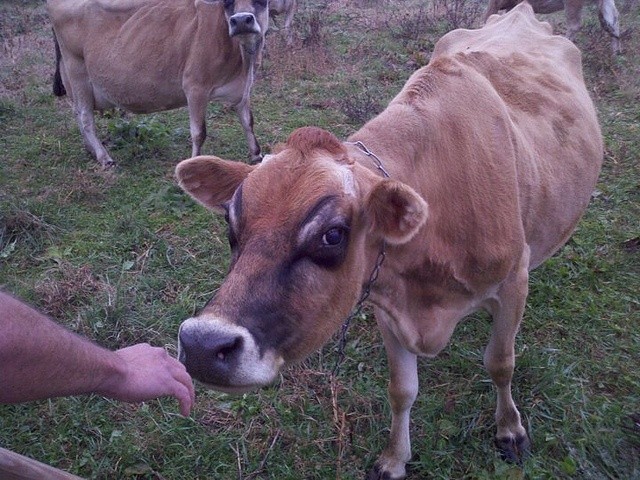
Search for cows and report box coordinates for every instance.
[479,1,622,59]
[268,0,297,46]
[174,1,605,480]
[46,0,269,168]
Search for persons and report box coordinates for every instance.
[0,290,195,418]
